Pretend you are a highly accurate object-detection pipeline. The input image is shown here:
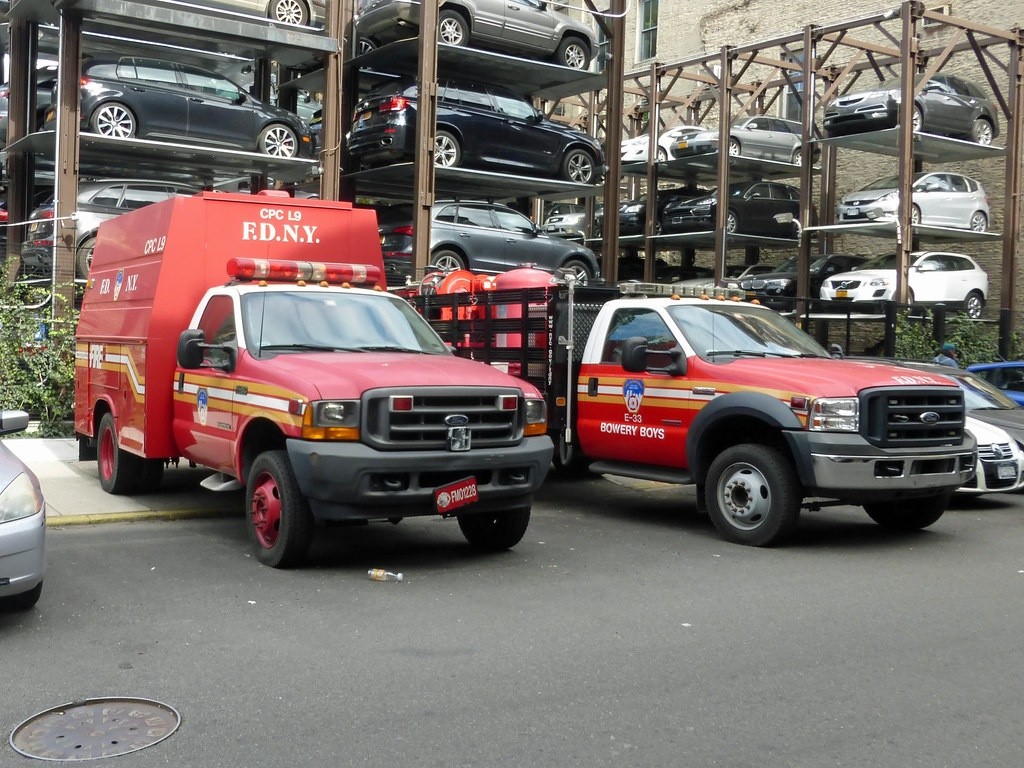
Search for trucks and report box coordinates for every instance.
[74,191,557,571]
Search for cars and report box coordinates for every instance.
[662,181,819,241]
[0,184,56,249]
[668,263,780,294]
[544,201,604,238]
[955,417,1024,501]
[135,0,317,29]
[837,171,991,232]
[966,362,1024,406]
[671,114,821,168]
[819,251,988,318]
[822,72,1000,146]
[842,355,1023,456]
[0,67,56,142]
[621,124,709,164]
[354,0,601,71]
[738,253,878,313]
[596,187,706,234]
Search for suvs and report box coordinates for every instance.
[20,179,204,282]
[372,199,601,288]
[345,72,606,186]
[41,55,317,160]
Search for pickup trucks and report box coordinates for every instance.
[407,270,980,550]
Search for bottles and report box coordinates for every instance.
[368,568,403,582]
